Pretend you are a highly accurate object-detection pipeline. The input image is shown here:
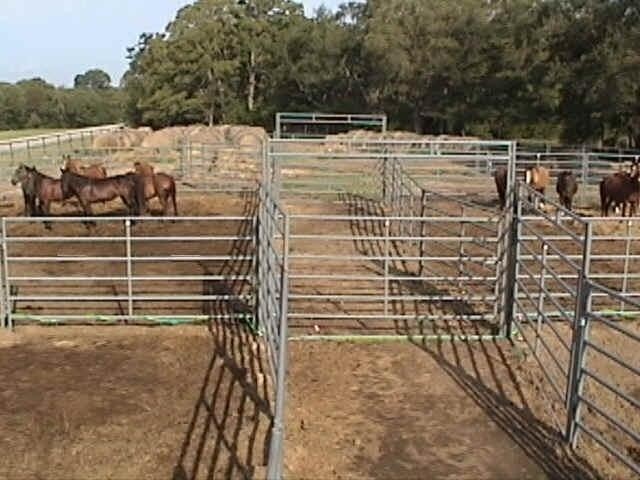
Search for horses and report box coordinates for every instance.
[492,166,510,207]
[556,170,578,212]
[523,165,549,212]
[10,153,178,232]
[599,157,640,223]
